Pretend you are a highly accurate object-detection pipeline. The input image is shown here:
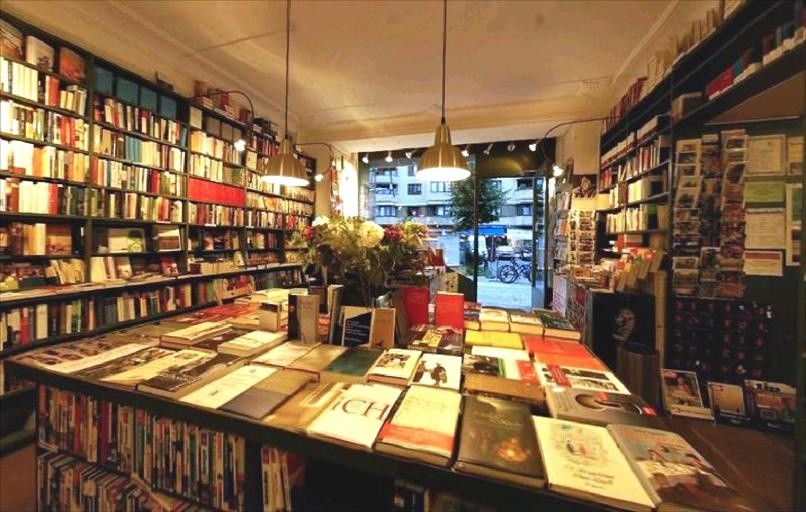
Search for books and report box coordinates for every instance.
[743,380,796,433]
[37,384,306,512]
[263,246,754,510]
[1,21,316,389]
[551,1,805,256]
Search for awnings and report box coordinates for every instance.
[468,225,507,234]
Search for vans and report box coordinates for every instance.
[466,235,488,260]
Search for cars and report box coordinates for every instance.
[520,247,533,260]
[495,245,515,260]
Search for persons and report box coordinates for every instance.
[486,235,510,260]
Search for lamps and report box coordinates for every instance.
[414,1,472,183]
[259,0,312,188]
[542,116,622,177]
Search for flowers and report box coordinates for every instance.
[289,215,438,308]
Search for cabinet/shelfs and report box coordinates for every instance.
[4,286,755,512]
[592,1,806,271]
[0,7,316,459]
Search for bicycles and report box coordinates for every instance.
[497,256,532,283]
